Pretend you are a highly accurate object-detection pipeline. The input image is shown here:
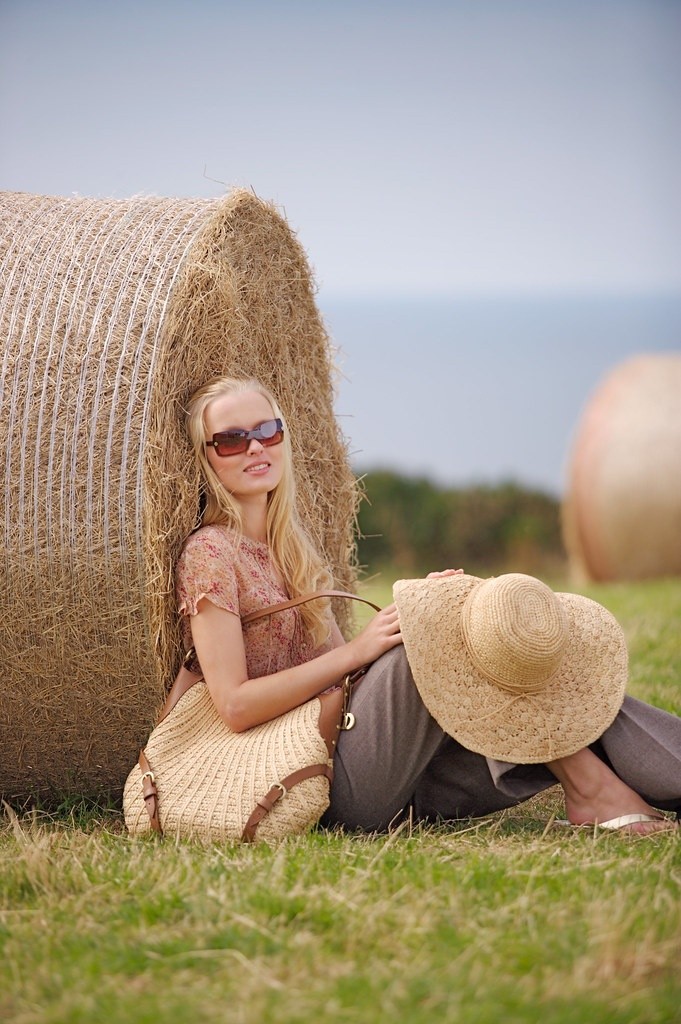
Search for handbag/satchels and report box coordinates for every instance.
[123,589,383,854]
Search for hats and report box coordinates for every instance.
[393,572,629,765]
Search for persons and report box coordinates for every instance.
[173,376,681,837]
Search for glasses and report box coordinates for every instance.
[205,418,285,457]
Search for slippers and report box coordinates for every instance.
[554,803,681,841]
